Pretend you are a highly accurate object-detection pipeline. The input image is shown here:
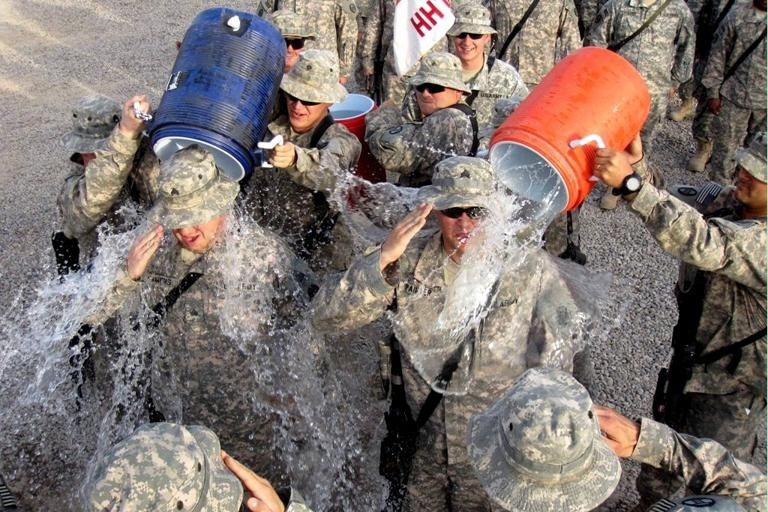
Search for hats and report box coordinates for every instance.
[736,132,767,185]
[408,51,472,96]
[279,48,348,103]
[411,156,500,210]
[464,365,622,512]
[61,95,121,153]
[447,2,498,37]
[67,422,243,512]
[146,143,241,230]
[264,10,316,40]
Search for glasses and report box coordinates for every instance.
[415,82,445,94]
[456,32,483,40]
[440,206,487,221]
[284,38,304,51]
[287,94,320,106]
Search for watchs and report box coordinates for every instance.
[611,170,642,195]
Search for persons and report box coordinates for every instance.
[590,130,767,512]
[468,365,767,512]
[365,51,480,190]
[701,0,766,184]
[384,2,533,145]
[54,91,164,442]
[587,2,698,210]
[310,157,576,512]
[688,1,736,173]
[482,1,585,95]
[348,1,381,103]
[238,50,361,305]
[253,1,356,88]
[75,146,312,511]
[81,420,315,512]
[268,9,351,117]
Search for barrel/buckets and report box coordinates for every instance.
[489,46,650,211]
[328,93,378,145]
[133,7,288,186]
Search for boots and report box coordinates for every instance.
[599,185,622,209]
[667,96,693,122]
[687,140,710,173]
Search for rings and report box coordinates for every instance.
[596,165,601,170]
